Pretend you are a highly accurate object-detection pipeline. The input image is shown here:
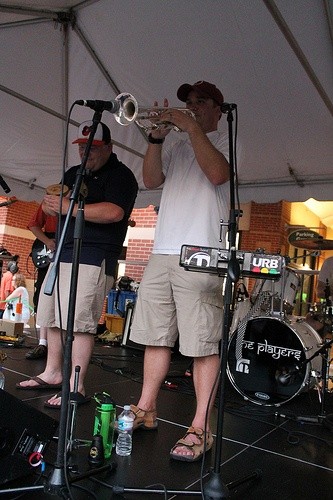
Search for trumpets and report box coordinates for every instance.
[112,92,198,133]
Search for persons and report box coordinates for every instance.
[15,120,141,409]
[108,79,236,463]
[26,194,57,360]
[0,261,30,324]
[317,256,333,305]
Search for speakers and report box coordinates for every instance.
[0,388,60,483]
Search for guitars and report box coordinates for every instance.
[31,230,58,269]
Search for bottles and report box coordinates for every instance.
[92,391,117,459]
[115,405,135,457]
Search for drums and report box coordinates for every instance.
[224,314,325,408]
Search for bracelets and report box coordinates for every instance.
[147,132,165,144]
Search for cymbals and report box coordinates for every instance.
[293,269,322,276]
[250,295,294,312]
[294,239,333,250]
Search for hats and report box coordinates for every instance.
[176,81,225,109]
[73,119,112,146]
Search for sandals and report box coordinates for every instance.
[111,404,159,430]
[169,425,213,463]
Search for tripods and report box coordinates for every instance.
[112,108,264,500]
[0,110,120,500]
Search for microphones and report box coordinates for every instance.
[220,101,238,114]
[76,99,120,113]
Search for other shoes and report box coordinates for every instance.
[25,344,48,360]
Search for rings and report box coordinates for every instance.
[170,114,173,118]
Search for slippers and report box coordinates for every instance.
[16,376,63,389]
[42,389,91,410]
